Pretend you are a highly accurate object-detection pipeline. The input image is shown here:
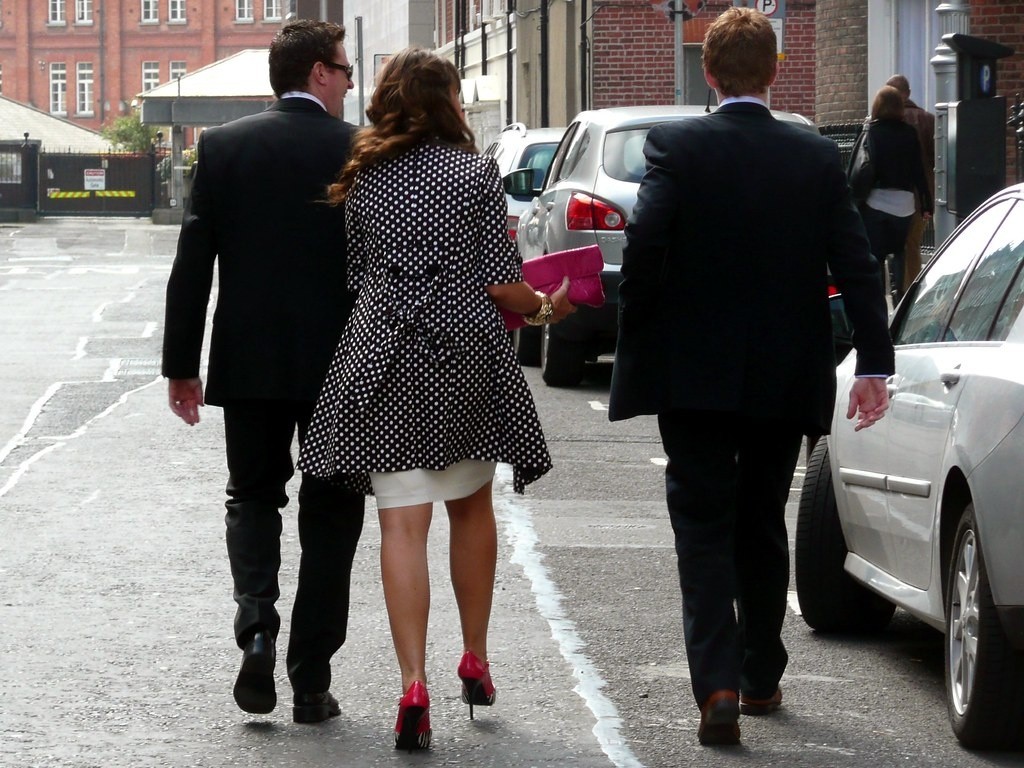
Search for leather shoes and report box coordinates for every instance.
[293,690,341,723]
[695,690,742,745]
[233,630,277,714]
[742,688,783,715]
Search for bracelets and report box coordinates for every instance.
[524,291,554,325]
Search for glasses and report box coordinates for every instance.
[322,60,353,81]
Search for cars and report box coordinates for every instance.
[795,180,1024,756]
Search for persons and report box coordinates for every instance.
[159,23,368,722]
[885,74,935,295]
[851,86,931,309]
[295,48,579,750]
[608,9,896,746]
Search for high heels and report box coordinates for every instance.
[458,651,496,721]
[395,681,433,754]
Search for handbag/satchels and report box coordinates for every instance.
[499,244,605,331]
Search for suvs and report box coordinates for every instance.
[483,121,569,255]
[513,103,850,388]
